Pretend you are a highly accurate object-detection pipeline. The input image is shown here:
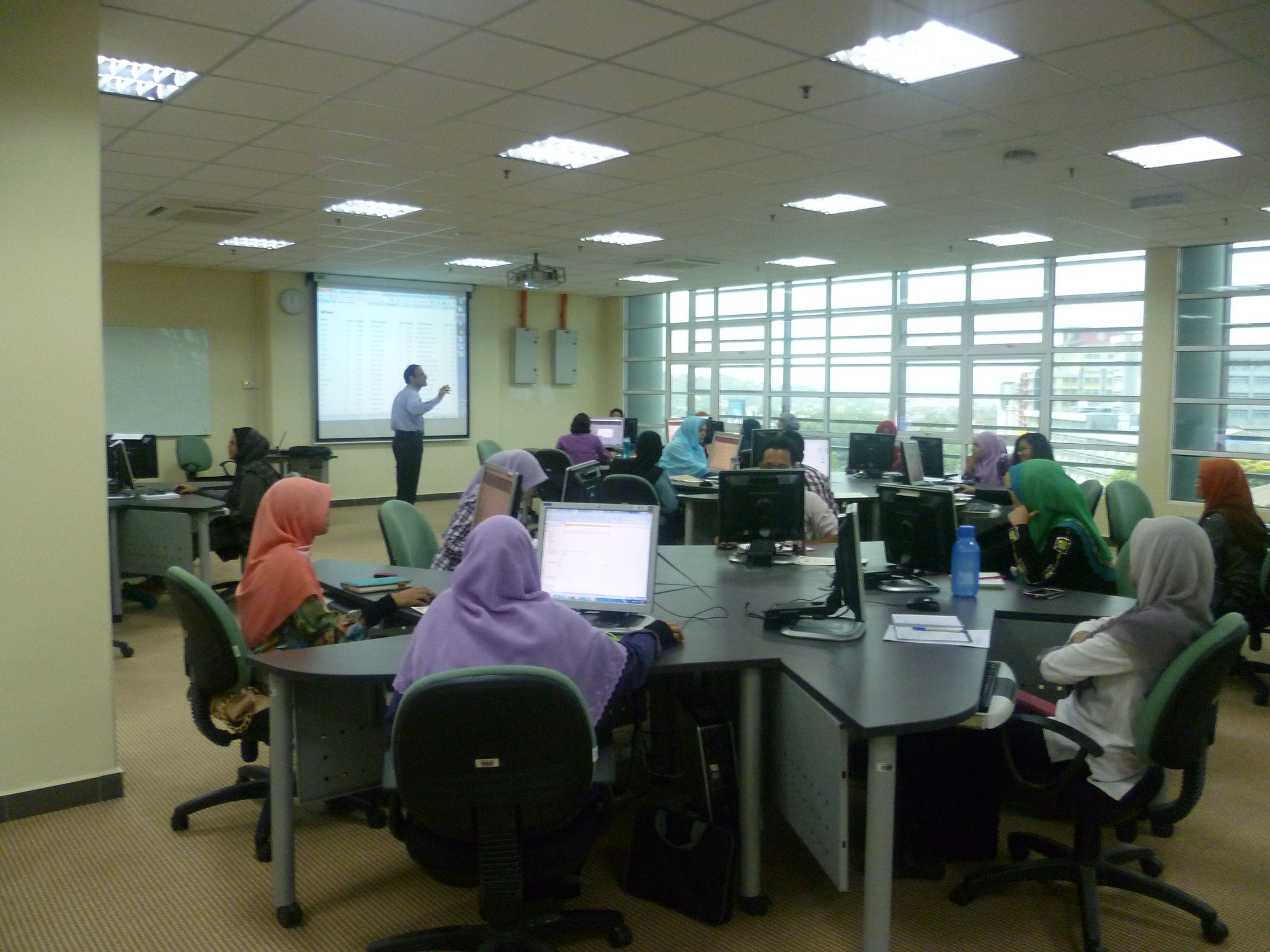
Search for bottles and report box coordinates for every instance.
[730,457,739,470]
[622,437,632,459]
[951,526,981,597]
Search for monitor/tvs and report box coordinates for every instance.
[106,434,159,479]
[589,417,637,451]
[535,501,661,634]
[848,432,943,486]
[561,460,604,504]
[763,503,865,642]
[470,462,524,532]
[108,441,136,492]
[876,482,959,579]
[704,419,830,567]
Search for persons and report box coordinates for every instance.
[919,516,1216,865]
[1196,458,1270,618]
[1009,459,1112,593]
[1014,433,1054,464]
[778,413,800,430]
[778,431,839,517]
[236,475,437,696]
[876,421,903,473]
[954,431,1012,507]
[387,513,684,790]
[391,364,450,504]
[121,427,280,610]
[616,430,680,545]
[757,442,839,547]
[433,448,548,572]
[556,413,613,465]
[610,409,632,451]
[658,416,721,478]
[740,419,760,451]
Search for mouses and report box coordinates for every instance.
[175,487,188,495]
[953,487,964,493]
[717,542,738,550]
[907,597,941,613]
[699,481,712,487]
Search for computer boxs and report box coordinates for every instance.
[664,686,740,823]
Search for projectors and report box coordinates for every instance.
[515,269,557,290]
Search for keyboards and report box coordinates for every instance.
[975,662,1001,715]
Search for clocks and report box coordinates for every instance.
[280,289,307,314]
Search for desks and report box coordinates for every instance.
[262,455,338,486]
[108,481,233,623]
[598,449,1013,545]
[309,541,1138,952]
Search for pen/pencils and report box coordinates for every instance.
[943,478,954,480]
[911,627,968,633]
[977,575,1009,579]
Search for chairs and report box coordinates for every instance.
[378,500,439,570]
[603,474,659,507]
[949,479,1270,952]
[530,449,582,537]
[176,435,238,482]
[367,666,632,952]
[476,440,502,465]
[166,566,387,862]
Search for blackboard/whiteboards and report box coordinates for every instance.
[105,326,212,437]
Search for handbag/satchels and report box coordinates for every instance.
[626,802,738,922]
[209,691,269,732]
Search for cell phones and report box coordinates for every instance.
[1023,588,1064,599]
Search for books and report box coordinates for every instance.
[342,576,412,594]
[891,614,972,642]
[978,572,1005,589]
